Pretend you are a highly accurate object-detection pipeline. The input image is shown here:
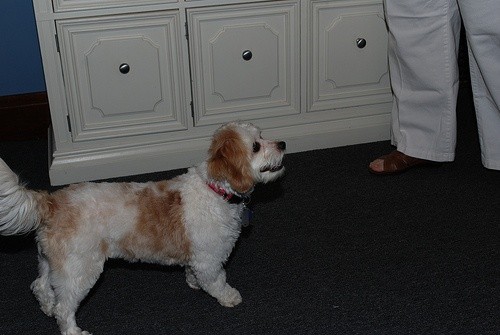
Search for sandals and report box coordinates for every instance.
[369,149,430,175]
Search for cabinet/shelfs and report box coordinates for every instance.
[32,0,395,187]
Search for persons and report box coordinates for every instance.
[368,1,500,179]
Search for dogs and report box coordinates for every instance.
[0,121,286,335]
